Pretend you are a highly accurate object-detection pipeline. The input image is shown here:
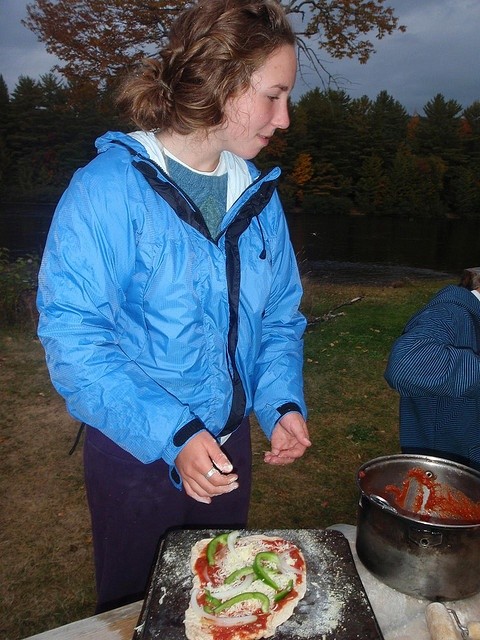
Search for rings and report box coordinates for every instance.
[205,467,216,480]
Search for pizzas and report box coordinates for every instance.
[186,533,306,639]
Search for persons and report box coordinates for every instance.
[383,267,480,471]
[34,1,313,615]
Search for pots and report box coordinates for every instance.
[354,452,480,604]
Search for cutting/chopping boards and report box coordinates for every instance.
[128,523,383,640]
